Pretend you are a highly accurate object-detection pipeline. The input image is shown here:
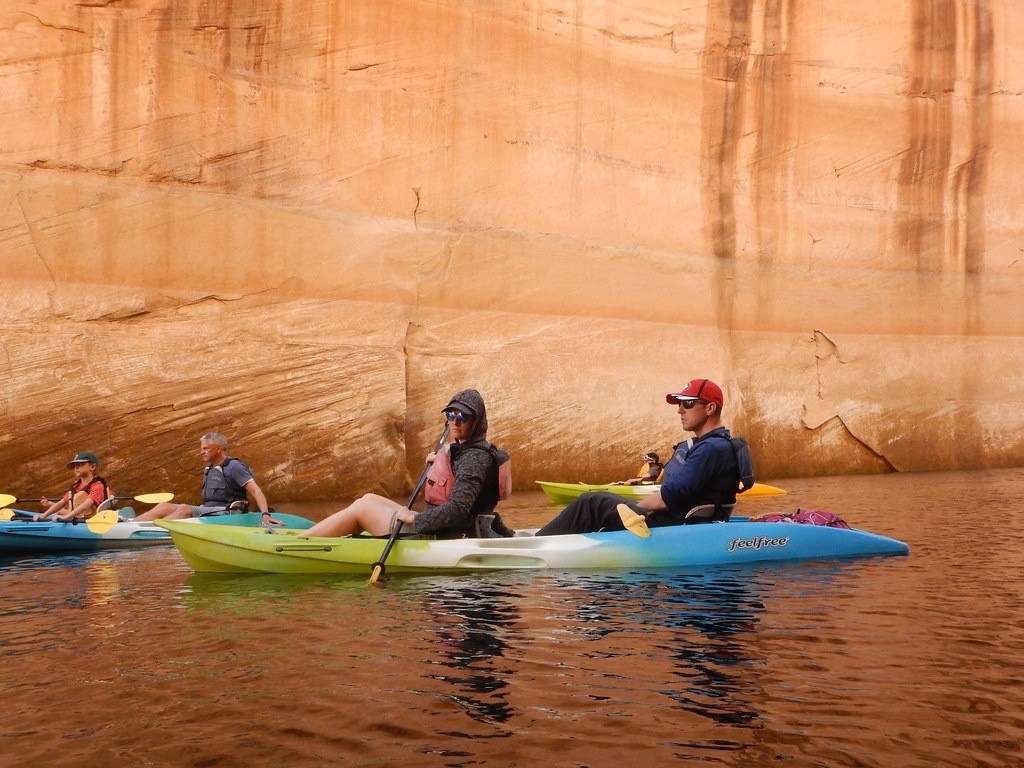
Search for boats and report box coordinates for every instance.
[6,505,138,523]
[0,510,319,552]
[153,518,910,577]
[534,478,787,507]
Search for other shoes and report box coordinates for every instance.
[475,514,503,538]
[491,512,516,538]
[265,528,277,535]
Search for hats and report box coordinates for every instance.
[665,379,724,408]
[66,452,99,469]
[441,401,475,415]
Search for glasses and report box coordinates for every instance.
[447,411,470,423]
[678,400,709,408]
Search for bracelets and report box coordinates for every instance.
[261,512,270,516]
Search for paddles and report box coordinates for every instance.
[0,492,174,535]
[369,425,451,583]
[615,502,676,539]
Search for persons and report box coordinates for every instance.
[33,452,114,522]
[118,432,286,526]
[298,389,510,540]
[535,379,756,537]
